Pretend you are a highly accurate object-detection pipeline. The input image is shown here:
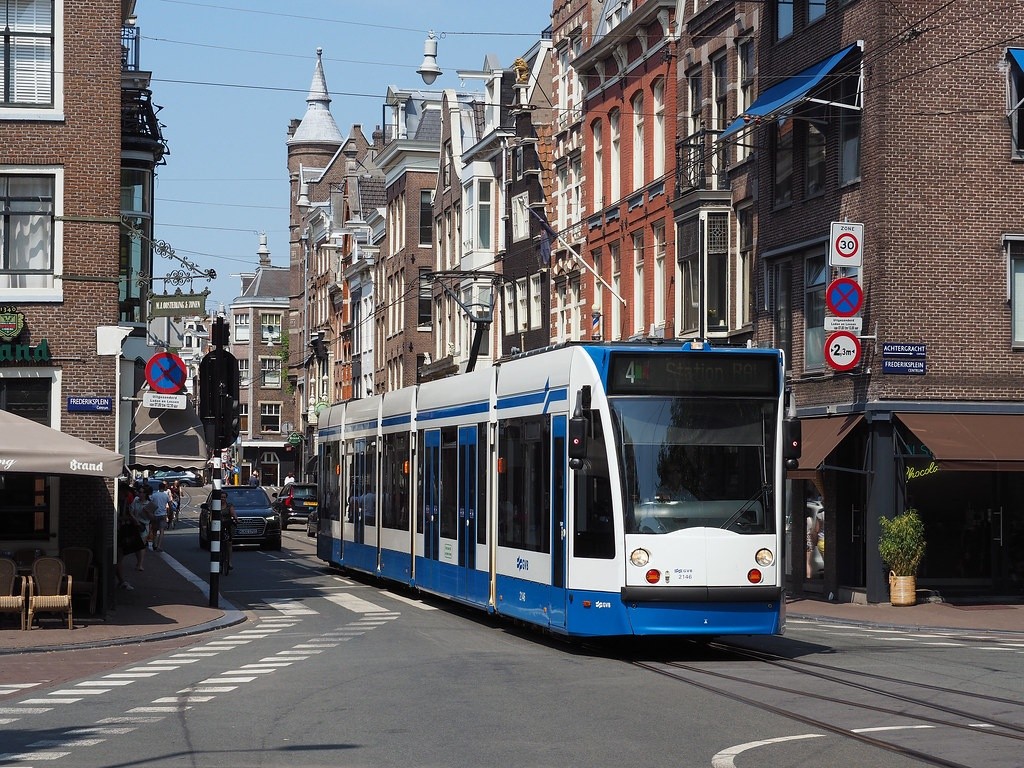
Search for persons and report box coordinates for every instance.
[655,463,702,502]
[318,488,401,524]
[114,470,183,592]
[248,470,260,487]
[220,491,244,570]
[283,472,295,487]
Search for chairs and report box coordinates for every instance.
[61,547,98,614]
[0,558,26,630]
[27,557,73,629]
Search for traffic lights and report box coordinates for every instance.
[226,396,239,443]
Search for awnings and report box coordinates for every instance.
[304,454,318,475]
[0,409,125,478]
[888,410,1024,473]
[127,388,208,471]
[785,409,872,481]
[714,39,863,142]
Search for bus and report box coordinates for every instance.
[318,198,801,645]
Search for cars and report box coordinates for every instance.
[135,469,204,488]
[307,506,318,537]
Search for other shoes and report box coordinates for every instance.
[120,580,134,590]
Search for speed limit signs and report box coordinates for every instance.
[836,233,858,258]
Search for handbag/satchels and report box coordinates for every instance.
[119,519,144,555]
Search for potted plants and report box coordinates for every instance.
[878,509,926,607]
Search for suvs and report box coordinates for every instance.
[198,485,281,550]
[271,482,318,530]
